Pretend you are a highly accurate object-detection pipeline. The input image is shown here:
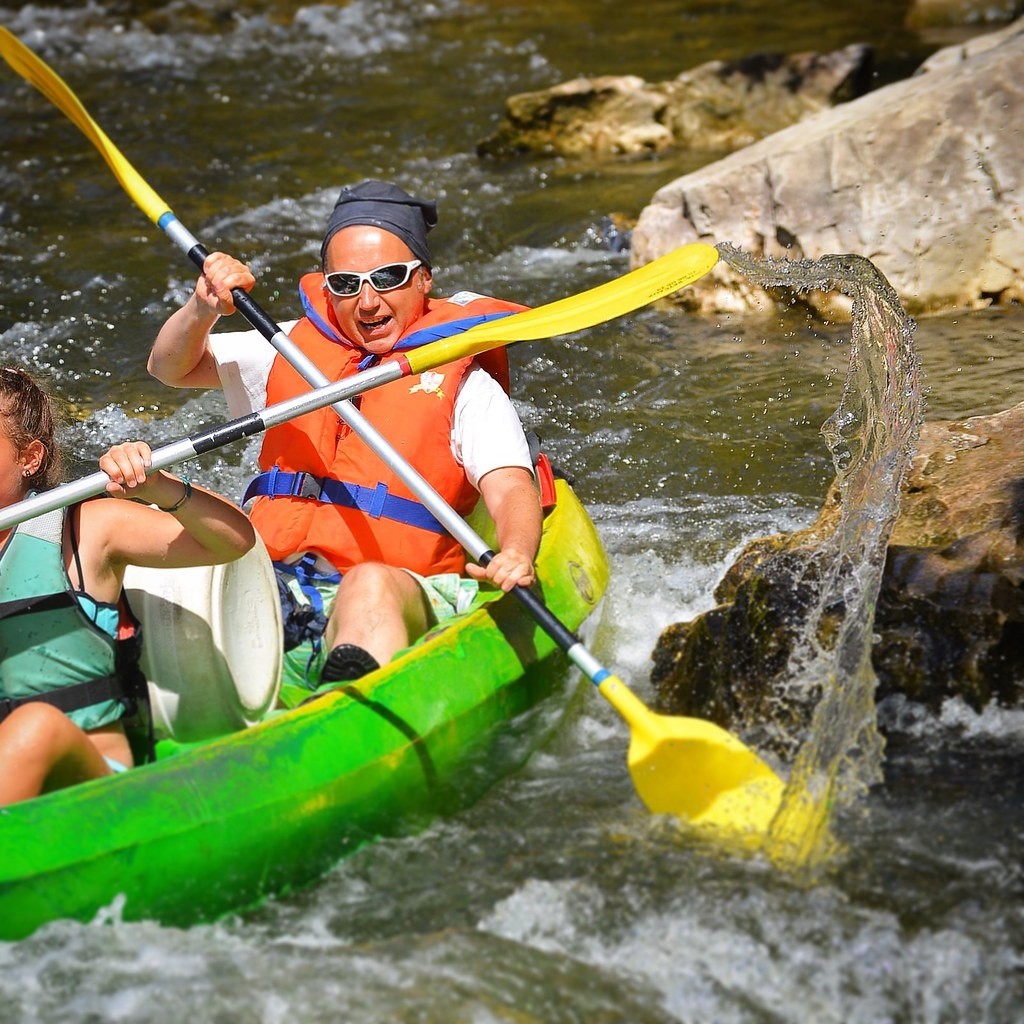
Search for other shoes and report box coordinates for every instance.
[318,644,381,685]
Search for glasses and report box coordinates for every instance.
[324,259,424,296]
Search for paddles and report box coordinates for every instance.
[0,241,721,532]
[0,24,784,835]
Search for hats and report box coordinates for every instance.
[320,181,438,273]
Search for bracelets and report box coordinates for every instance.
[156,474,192,513]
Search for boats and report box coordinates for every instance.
[0,452,615,958]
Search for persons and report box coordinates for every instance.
[0,363,256,823]
[144,178,545,692]
